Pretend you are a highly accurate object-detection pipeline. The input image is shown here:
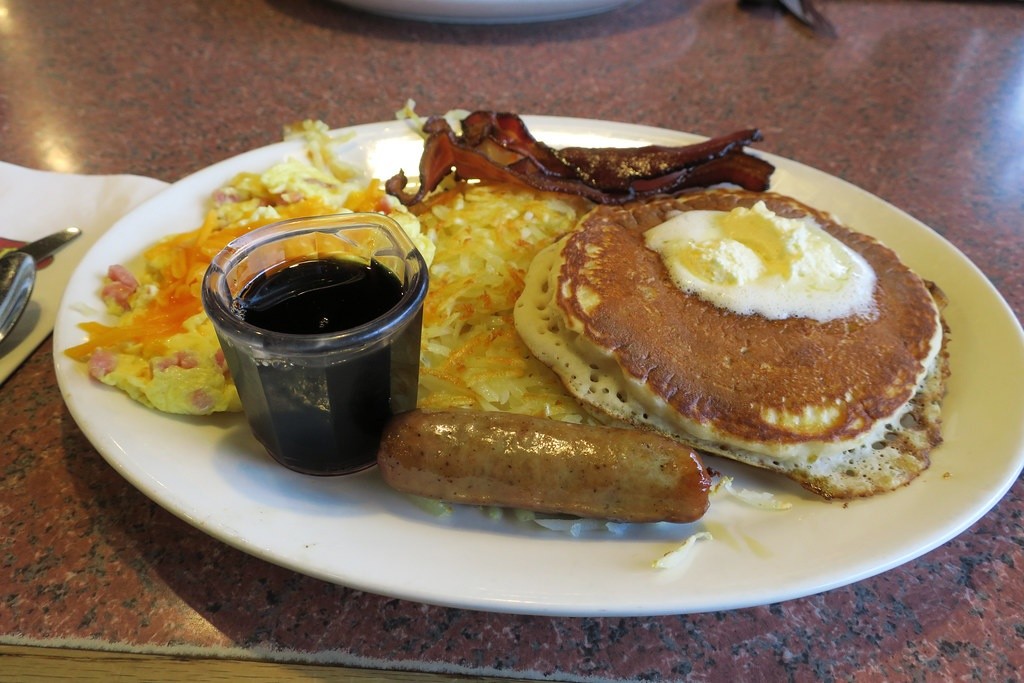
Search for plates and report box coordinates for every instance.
[49,112,1024,618]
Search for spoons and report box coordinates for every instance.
[0,250,37,344]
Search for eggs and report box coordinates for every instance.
[647,207,873,315]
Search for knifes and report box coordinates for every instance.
[781,0,838,41]
[0,226,82,264]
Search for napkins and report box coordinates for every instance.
[1,159,176,389]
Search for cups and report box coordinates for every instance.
[339,0,624,25]
[198,209,433,476]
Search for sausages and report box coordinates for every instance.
[379,412,710,522]
